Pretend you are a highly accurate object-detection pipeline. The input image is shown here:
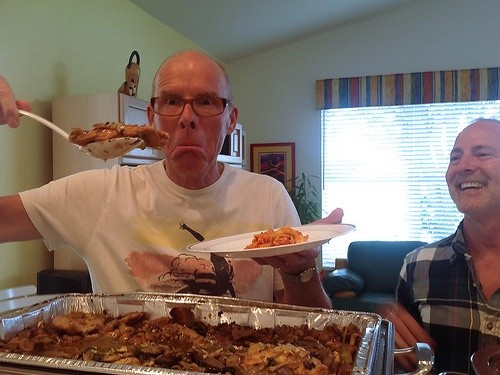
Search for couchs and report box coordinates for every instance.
[322,241,429,314]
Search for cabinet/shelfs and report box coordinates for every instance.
[52,93,243,272]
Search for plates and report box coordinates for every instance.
[186,224,356,258]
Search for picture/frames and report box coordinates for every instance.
[250,143,296,199]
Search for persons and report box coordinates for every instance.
[0,49,345,309]
[380,116,500,375]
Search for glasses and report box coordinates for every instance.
[151,96,232,117]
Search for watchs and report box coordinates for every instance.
[278,267,316,284]
